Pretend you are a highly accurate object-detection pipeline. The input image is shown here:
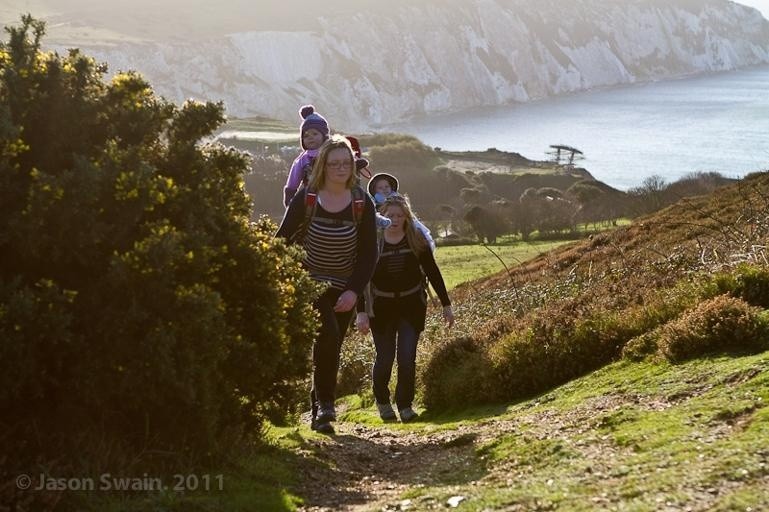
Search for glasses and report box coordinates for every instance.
[326,161,354,170]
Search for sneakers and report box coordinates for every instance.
[314,400,336,423]
[311,412,335,434]
[376,400,396,421]
[399,405,418,423]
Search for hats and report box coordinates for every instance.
[300,105,329,136]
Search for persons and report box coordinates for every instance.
[367,172,399,228]
[283,105,331,208]
[273,135,379,433]
[356,197,454,421]
